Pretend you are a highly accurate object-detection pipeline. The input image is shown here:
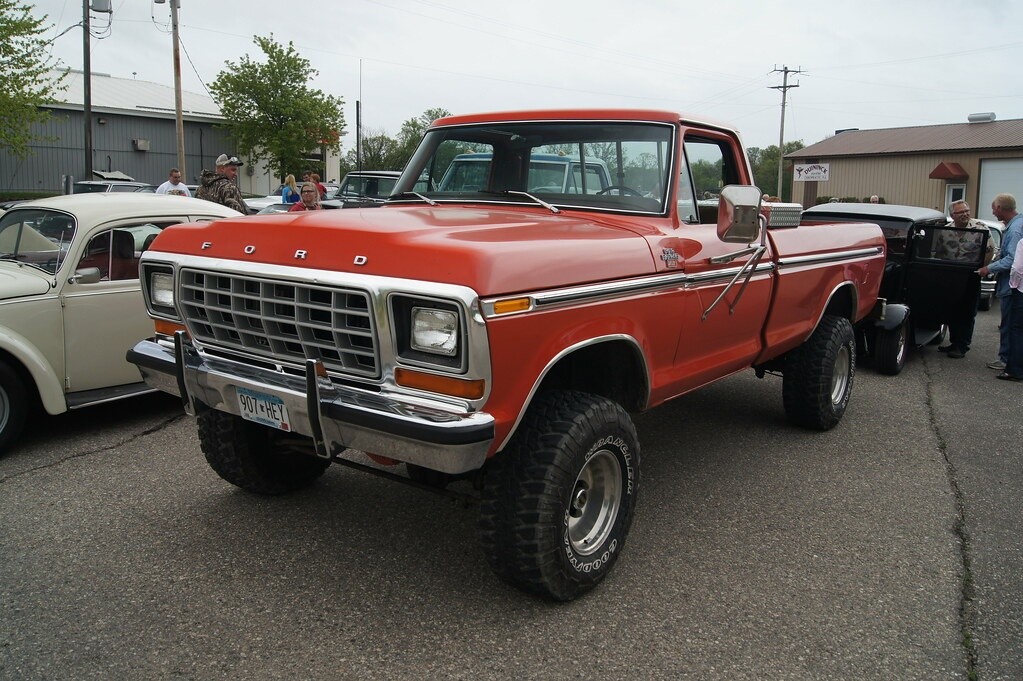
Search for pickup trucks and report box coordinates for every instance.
[124,105,889,605]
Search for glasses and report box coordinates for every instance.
[303,175,309,179]
[301,190,314,193]
[872,201,878,203]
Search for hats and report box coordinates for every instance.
[215,153,244,166]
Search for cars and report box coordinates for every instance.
[435,149,614,198]
[945,216,1006,310]
[70,168,438,217]
[796,203,991,375]
[0,188,251,450]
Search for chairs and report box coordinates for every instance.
[78,253,127,282]
[120,259,141,280]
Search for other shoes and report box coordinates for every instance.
[938,343,954,352]
[948,347,966,358]
[985,359,1007,369]
[996,371,1023,381]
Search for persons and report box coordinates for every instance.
[976,192,1023,369]
[868,195,880,204]
[155,168,192,198]
[932,201,994,360]
[282,171,327,215]
[996,237,1023,381]
[194,152,251,217]
[699,192,721,202]
[762,193,781,203]
